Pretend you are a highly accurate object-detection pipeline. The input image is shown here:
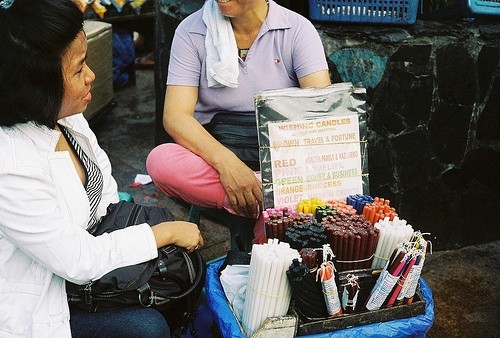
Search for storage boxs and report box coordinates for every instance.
[203,258,433,338]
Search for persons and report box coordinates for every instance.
[146,0,331,253]
[0,0,206,338]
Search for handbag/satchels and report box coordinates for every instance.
[202,112,260,171]
[66,200,206,312]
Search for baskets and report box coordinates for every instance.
[467,0,500,15]
[310,0,419,26]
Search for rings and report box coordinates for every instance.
[195,245,201,252]
